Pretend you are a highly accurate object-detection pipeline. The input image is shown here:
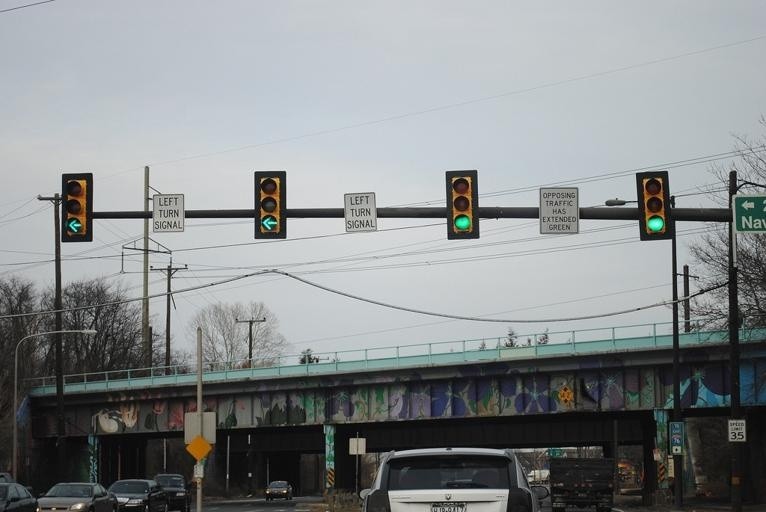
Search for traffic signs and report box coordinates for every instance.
[545,448,568,459]
[729,193,766,237]
[150,194,187,234]
[342,192,381,233]
[726,418,746,444]
[669,421,685,455]
[537,187,582,237]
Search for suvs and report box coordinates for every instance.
[360,444,549,512]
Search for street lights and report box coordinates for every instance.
[11,325,103,483]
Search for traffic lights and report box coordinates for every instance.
[253,171,284,239]
[631,171,676,241]
[60,171,93,243]
[445,167,481,241]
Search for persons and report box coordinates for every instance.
[299,349,314,363]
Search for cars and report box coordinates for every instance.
[0,470,195,512]
[265,479,294,499]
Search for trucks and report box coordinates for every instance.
[546,456,605,511]
[527,469,549,484]
